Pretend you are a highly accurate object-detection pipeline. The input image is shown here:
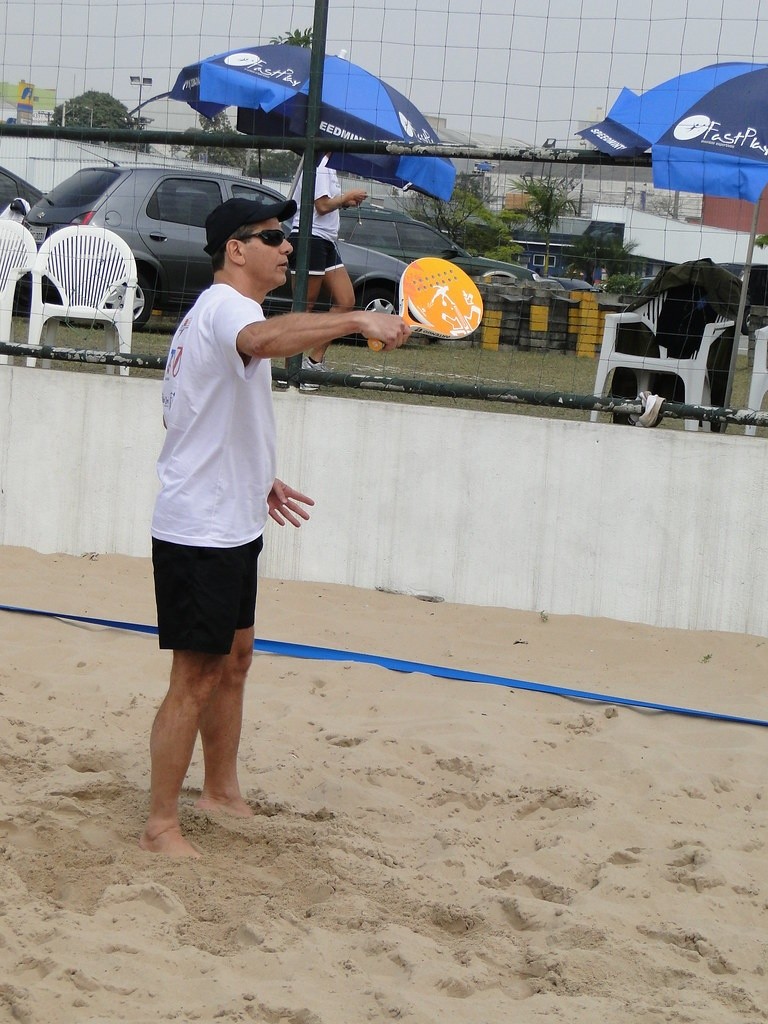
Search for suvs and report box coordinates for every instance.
[0,146,410,346]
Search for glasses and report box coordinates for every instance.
[234,229,287,246]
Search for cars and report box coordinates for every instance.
[617,262,768,336]
[336,203,544,281]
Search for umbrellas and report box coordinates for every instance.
[168,46,457,227]
[573,62,768,435]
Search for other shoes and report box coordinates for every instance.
[277,379,319,390]
[302,357,330,373]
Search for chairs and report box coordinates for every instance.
[589,258,768,435]
[0,219,137,376]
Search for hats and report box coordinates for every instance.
[203,198,297,258]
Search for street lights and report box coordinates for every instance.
[85,107,93,127]
[578,142,587,217]
[129,76,153,161]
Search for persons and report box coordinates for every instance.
[138,198,412,858]
[275,153,368,391]
